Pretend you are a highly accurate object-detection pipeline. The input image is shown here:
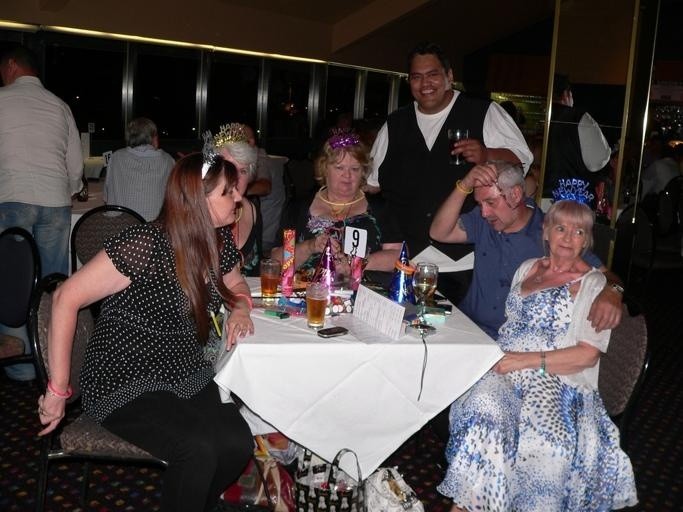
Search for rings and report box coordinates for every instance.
[40,408,44,415]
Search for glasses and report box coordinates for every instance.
[494,163,539,210]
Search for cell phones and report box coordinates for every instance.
[317,326,348,337]
[435,304,454,314]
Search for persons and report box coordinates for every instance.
[246,147,272,196]
[37,154,255,512]
[202,122,263,278]
[1,43,84,391]
[103,117,176,222]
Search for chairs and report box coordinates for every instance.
[585,298,650,464]
[26,271,174,512]
[64,205,148,275]
[0,223,46,397]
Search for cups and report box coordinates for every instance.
[305,282,328,330]
[446,129,468,165]
[258,258,280,302]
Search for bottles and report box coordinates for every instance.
[77,164,88,202]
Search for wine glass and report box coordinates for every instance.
[412,262,439,326]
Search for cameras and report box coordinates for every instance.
[406,323,436,338]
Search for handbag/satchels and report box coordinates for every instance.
[224,435,296,512]
[362,467,424,512]
[294,448,364,511]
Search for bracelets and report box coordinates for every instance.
[233,293,253,312]
[47,383,73,399]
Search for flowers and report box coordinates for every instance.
[306,214,333,236]
